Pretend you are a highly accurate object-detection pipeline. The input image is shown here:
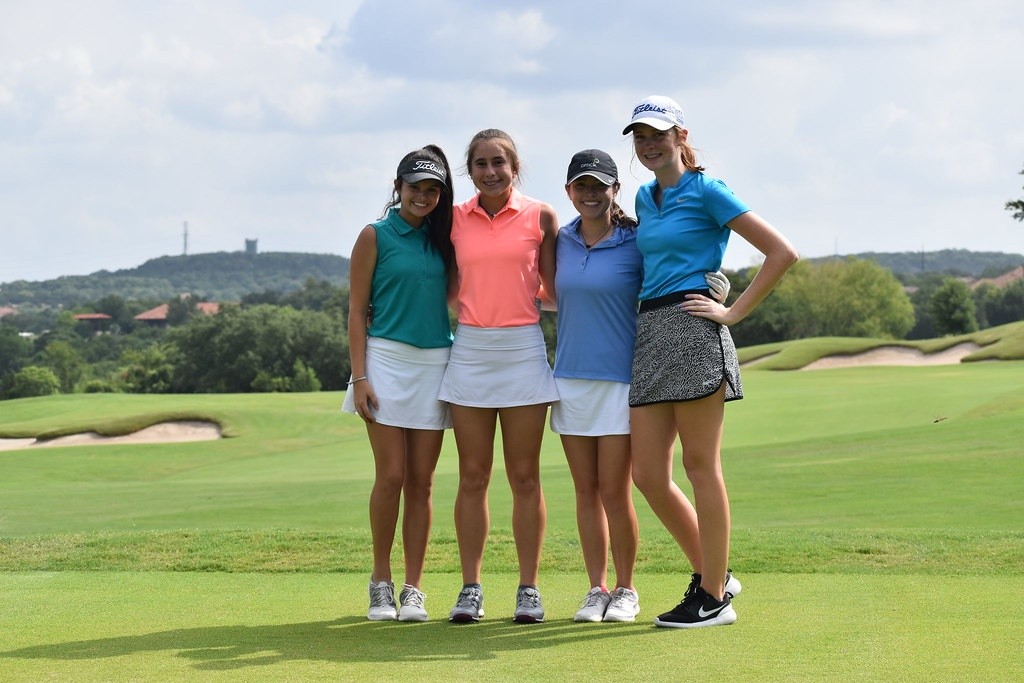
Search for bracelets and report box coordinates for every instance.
[345,377,367,385]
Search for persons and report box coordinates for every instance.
[622,96,798,628]
[437,129,559,623]
[537,148,730,621]
[343,143,458,621]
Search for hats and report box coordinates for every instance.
[622,95,682,134]
[565,148,617,186]
[397,158,447,187]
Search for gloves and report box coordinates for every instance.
[705,270,731,303]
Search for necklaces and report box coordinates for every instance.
[479,198,510,217]
[580,223,614,248]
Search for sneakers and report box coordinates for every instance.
[655,586,737,628]
[513,587,544,622]
[448,587,484,622]
[398,584,428,621]
[683,570,742,599]
[574,587,611,622]
[604,584,640,622]
[367,574,398,619]
